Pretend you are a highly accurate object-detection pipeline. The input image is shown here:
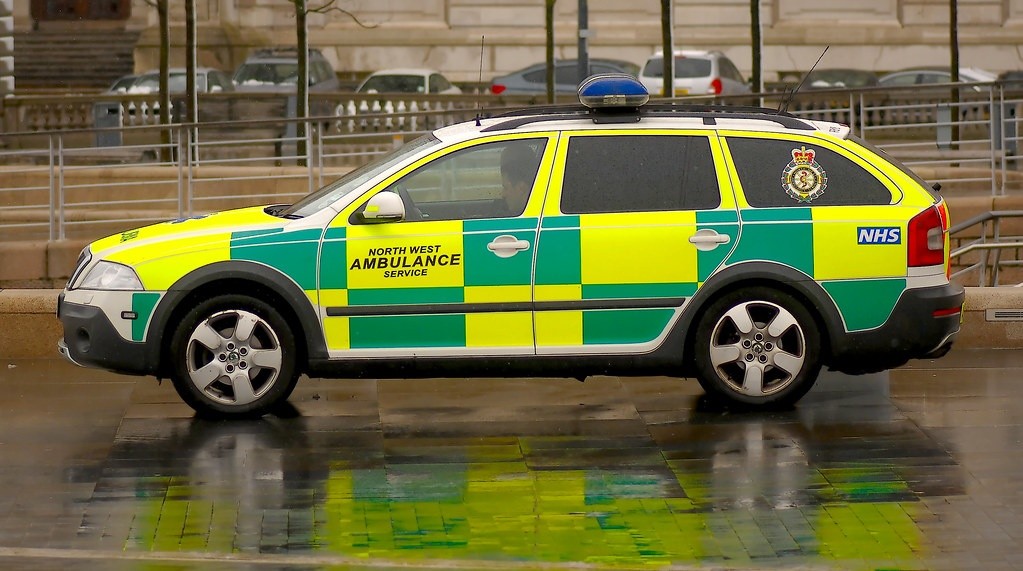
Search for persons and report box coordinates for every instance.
[472,145,537,217]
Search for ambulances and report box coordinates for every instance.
[55,71,967,415]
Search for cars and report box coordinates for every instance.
[106,64,234,93]
[792,69,884,111]
[876,65,998,94]
[332,69,465,133]
[492,58,642,107]
[987,70,1023,100]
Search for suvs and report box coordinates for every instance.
[230,45,334,119]
[637,48,766,117]
